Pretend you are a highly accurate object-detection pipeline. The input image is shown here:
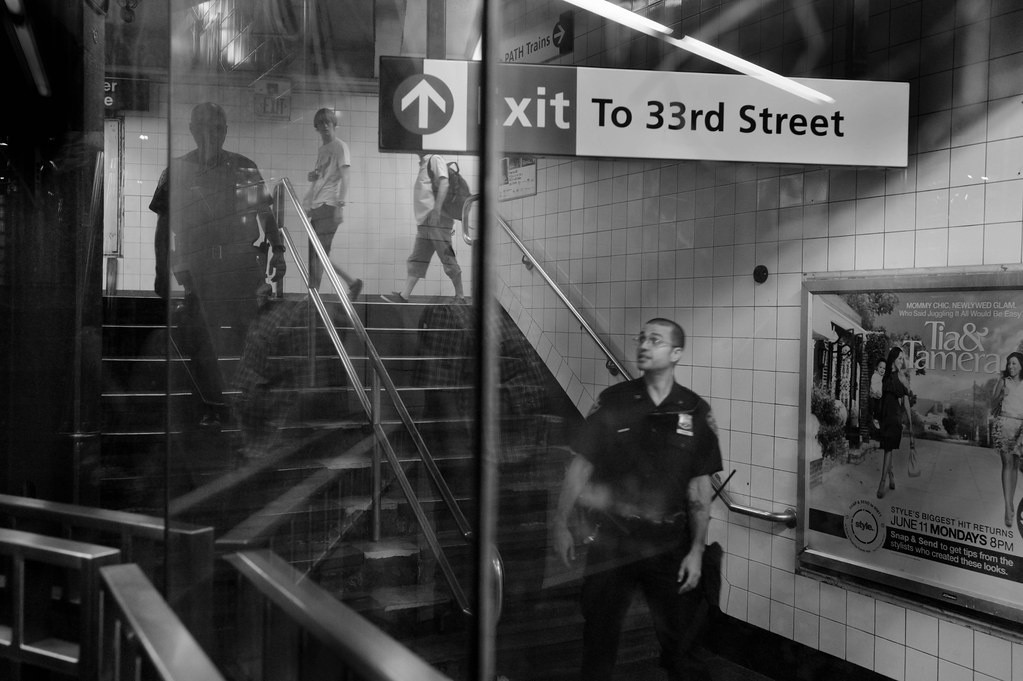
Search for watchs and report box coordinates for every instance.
[271,243,287,253]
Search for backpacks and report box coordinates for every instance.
[428,155,471,220]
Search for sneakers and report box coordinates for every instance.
[382,292,409,304]
[348,278,364,302]
[448,296,467,305]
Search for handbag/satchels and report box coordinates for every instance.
[908,443,922,477]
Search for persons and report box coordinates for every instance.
[417,291,544,679]
[230,288,335,556]
[550,316,724,681]
[293,107,366,303]
[868,357,887,442]
[148,102,287,436]
[990,350,1023,527]
[876,346,915,498]
[379,153,468,304]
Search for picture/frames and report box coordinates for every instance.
[793,265,1022,644]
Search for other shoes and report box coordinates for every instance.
[1003,507,1016,526]
[877,482,897,498]
